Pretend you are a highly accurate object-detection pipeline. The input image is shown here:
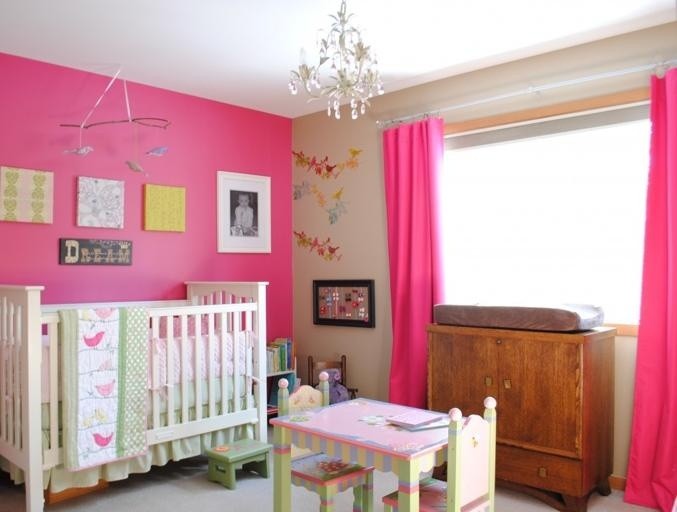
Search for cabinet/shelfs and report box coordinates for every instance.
[423,320,620,512]
[267,358,299,417]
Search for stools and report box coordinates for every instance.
[203,438,273,489]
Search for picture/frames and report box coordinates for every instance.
[215,170,274,254]
[311,278,378,329]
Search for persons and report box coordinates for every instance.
[230,192,254,237]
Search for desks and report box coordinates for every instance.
[266,394,469,512]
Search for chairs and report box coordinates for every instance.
[277,370,381,512]
[306,355,358,399]
[381,393,500,511]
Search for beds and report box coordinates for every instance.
[0,279,272,512]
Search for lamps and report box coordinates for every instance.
[287,0,388,119]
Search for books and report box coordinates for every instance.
[266,337,302,409]
[386,409,450,431]
